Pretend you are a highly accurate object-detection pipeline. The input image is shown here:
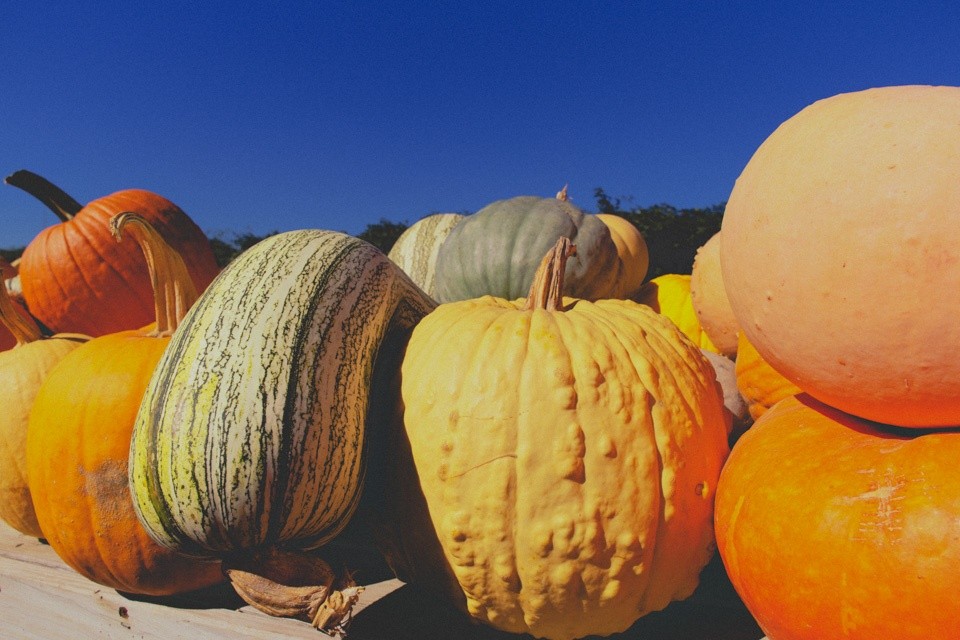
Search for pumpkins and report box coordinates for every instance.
[0,84,960,640]
